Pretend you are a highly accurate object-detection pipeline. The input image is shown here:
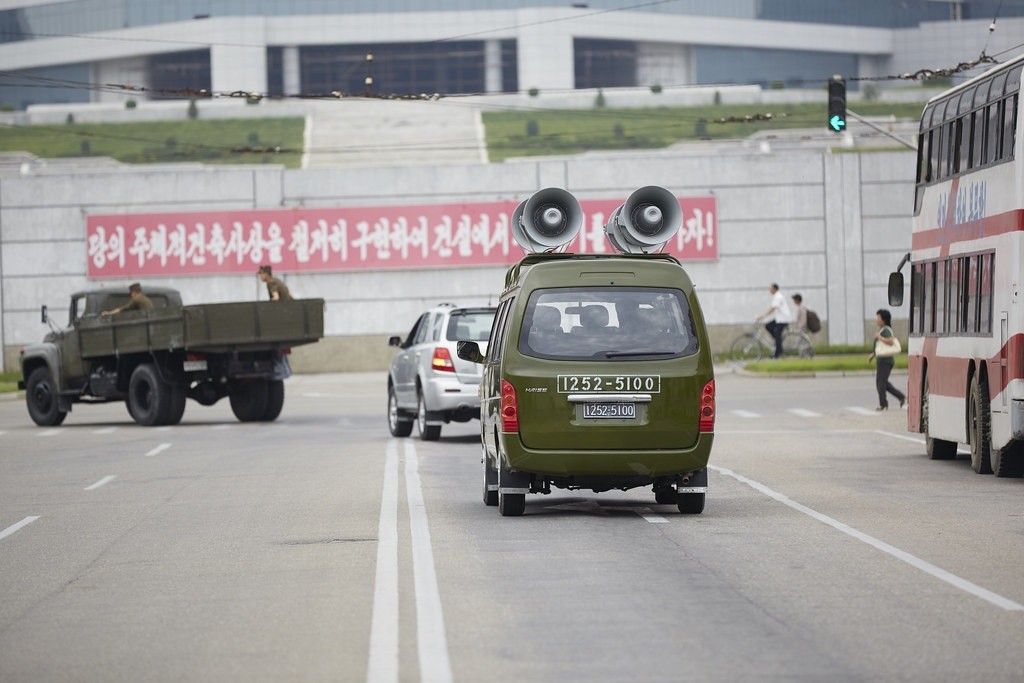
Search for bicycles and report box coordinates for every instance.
[729,319,815,367]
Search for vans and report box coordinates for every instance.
[455,186,717,517]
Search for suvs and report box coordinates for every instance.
[385,300,496,441]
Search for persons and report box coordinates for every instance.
[757,284,792,359]
[792,294,814,358]
[256,268,294,302]
[102,283,153,315]
[869,309,906,411]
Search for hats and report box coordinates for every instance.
[257,265,272,274]
[128,284,141,294]
[791,294,802,303]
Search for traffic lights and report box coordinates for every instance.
[827,74,846,132]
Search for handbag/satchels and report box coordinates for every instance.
[875,326,902,357]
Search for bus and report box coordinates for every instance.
[888,50,1024,481]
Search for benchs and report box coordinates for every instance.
[530,306,680,348]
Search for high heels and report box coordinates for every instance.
[876,401,889,411]
[900,396,907,407]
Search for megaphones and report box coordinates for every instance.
[510,187,582,254]
[608,185,682,255]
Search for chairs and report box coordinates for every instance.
[570,305,618,336]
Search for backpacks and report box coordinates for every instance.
[807,310,821,332]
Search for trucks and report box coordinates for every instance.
[16,283,325,427]
